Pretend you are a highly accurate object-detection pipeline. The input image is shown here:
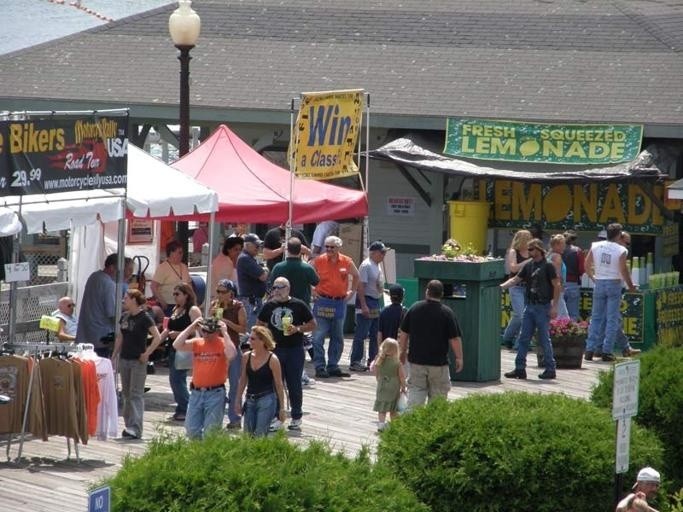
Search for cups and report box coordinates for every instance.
[213,308,222,320]
[281,317,291,337]
[580,251,679,290]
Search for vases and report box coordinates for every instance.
[535,336,585,371]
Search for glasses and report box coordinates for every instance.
[217,290,227,293]
[326,246,334,248]
[68,304,75,307]
[273,287,284,289]
[173,292,178,295]
[529,248,534,251]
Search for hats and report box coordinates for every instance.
[244,233,264,244]
[218,279,237,297]
[632,467,660,489]
[369,241,390,251]
[390,285,403,298]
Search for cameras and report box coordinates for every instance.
[100,332,115,345]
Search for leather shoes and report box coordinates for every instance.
[123,429,137,438]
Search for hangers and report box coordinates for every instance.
[0,341,111,370]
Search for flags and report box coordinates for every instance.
[287,88,366,182]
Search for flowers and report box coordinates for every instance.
[548,313,587,340]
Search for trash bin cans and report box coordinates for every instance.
[414,257,505,382]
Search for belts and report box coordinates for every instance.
[517,283,527,287]
[319,293,347,300]
[191,384,225,391]
[365,295,380,300]
[247,390,275,399]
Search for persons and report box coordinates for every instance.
[398,278,465,415]
[376,283,410,385]
[369,337,409,437]
[613,465,665,512]
[51,216,362,447]
[348,240,393,373]
[500,221,643,381]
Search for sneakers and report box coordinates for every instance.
[288,416,302,430]
[585,348,641,361]
[504,341,512,348]
[378,423,384,431]
[227,422,241,429]
[301,361,366,385]
[268,420,284,431]
[539,370,555,379]
[505,369,526,378]
